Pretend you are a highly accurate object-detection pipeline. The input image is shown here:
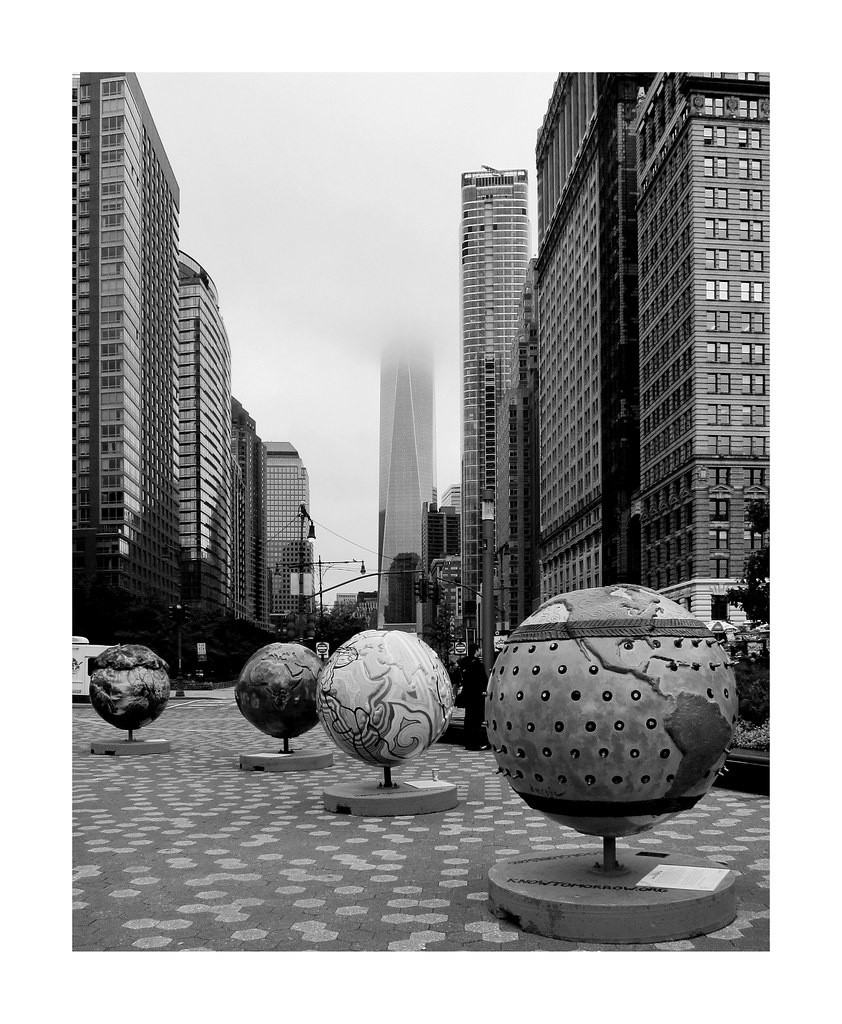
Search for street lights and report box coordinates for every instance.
[297,501,317,645]
[317,554,366,662]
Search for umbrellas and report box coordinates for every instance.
[704,620,738,641]
[751,623,770,638]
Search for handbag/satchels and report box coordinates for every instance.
[455,692,466,709]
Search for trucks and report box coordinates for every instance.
[72,636,119,703]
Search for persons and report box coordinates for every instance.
[751,647,770,670]
[458,644,491,751]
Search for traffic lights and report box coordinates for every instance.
[169,603,192,623]
[415,579,445,607]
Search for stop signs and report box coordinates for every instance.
[316,642,329,659]
[454,642,468,655]
